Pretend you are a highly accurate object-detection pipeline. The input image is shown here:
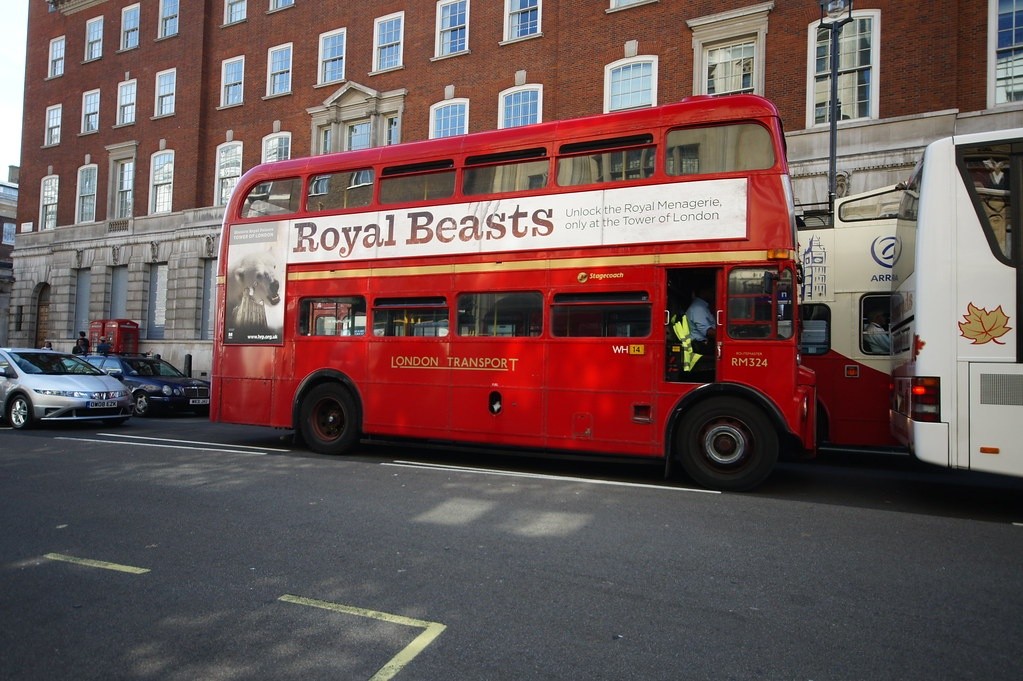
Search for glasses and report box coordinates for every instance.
[878,313,885,319]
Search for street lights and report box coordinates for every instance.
[819,0,855,226]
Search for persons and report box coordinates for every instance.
[41,341,52,351]
[72,339,88,354]
[97,336,112,353]
[974,157,1008,190]
[76,331,90,346]
[867,305,897,356]
[683,290,718,367]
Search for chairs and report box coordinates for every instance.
[676,309,715,381]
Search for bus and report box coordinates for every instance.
[888,128,1023,478]
[208,93,818,494]
[791,180,939,450]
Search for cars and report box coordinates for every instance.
[0,347,135,430]
[72,350,210,418]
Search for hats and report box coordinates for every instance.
[98,336,105,341]
